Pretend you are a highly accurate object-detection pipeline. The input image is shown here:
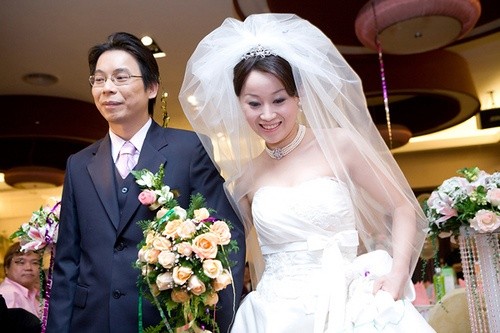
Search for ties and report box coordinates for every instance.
[115,141,137,179]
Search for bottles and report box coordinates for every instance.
[432,267,445,300]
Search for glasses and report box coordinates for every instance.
[88,70,145,88]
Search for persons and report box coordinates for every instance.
[0,242,45,333]
[45,32,246,333]
[178,14,436,333]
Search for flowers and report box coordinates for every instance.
[125,164,239,333]
[419,163,500,312]
[10,195,63,333]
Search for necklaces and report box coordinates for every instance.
[265,124,307,159]
